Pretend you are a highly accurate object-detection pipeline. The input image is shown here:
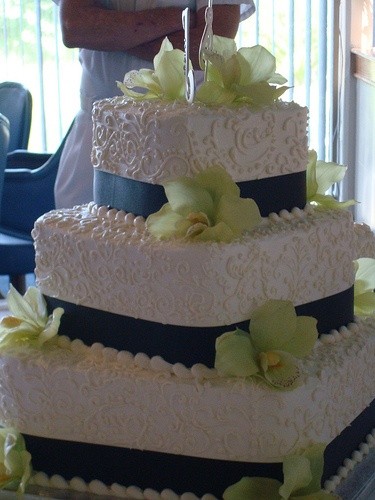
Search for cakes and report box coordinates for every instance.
[0,92,375,500]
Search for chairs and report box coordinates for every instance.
[0,81,74,299]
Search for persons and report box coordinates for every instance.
[50,0,256,212]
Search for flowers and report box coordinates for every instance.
[0,34,375,500]
